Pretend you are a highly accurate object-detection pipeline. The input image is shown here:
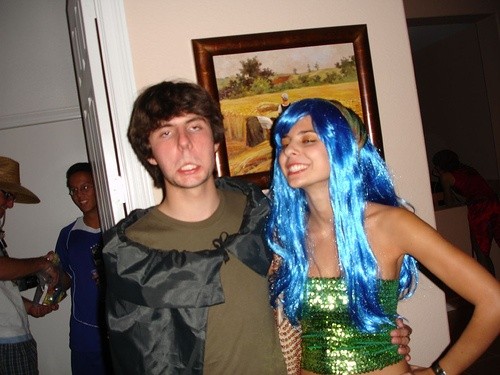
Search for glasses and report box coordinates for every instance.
[69,184,93,196]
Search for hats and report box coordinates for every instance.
[0,156,40,204]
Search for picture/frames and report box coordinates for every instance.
[191,23,386,189]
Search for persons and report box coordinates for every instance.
[100,80,294,375]
[0,156,71,375]
[39,163,103,375]
[432,149,500,276]
[263,99,500,375]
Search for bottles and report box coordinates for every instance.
[32,254,60,306]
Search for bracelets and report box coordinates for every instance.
[431,361,447,374]
